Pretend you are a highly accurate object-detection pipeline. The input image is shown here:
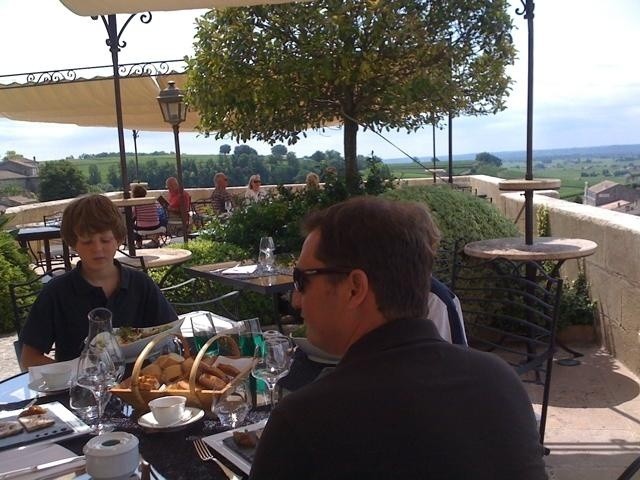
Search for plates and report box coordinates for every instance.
[202,420,268,476]
[138,407,205,432]
[0,400,92,455]
[28,378,74,393]
[1,361,77,405]
[222,265,258,274]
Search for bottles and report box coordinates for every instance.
[190,314,219,358]
[77,308,126,390]
[236,317,274,408]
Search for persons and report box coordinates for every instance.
[245,196,549,480]
[400,198,470,349]
[144,177,191,249]
[128,184,161,251]
[304,171,321,193]
[19,194,184,376]
[245,173,268,206]
[211,173,236,215]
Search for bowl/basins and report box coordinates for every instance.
[86,318,185,364]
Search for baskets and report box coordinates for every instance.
[110,332,258,413]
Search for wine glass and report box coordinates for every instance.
[77,351,116,436]
[260,237,275,264]
[251,343,291,412]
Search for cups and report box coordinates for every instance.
[148,396,187,425]
[213,377,248,430]
[269,336,295,373]
[258,251,275,272]
[42,365,72,388]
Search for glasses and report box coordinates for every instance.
[293,266,347,292]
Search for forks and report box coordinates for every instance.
[192,438,244,480]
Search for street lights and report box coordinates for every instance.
[155,81,189,247]
